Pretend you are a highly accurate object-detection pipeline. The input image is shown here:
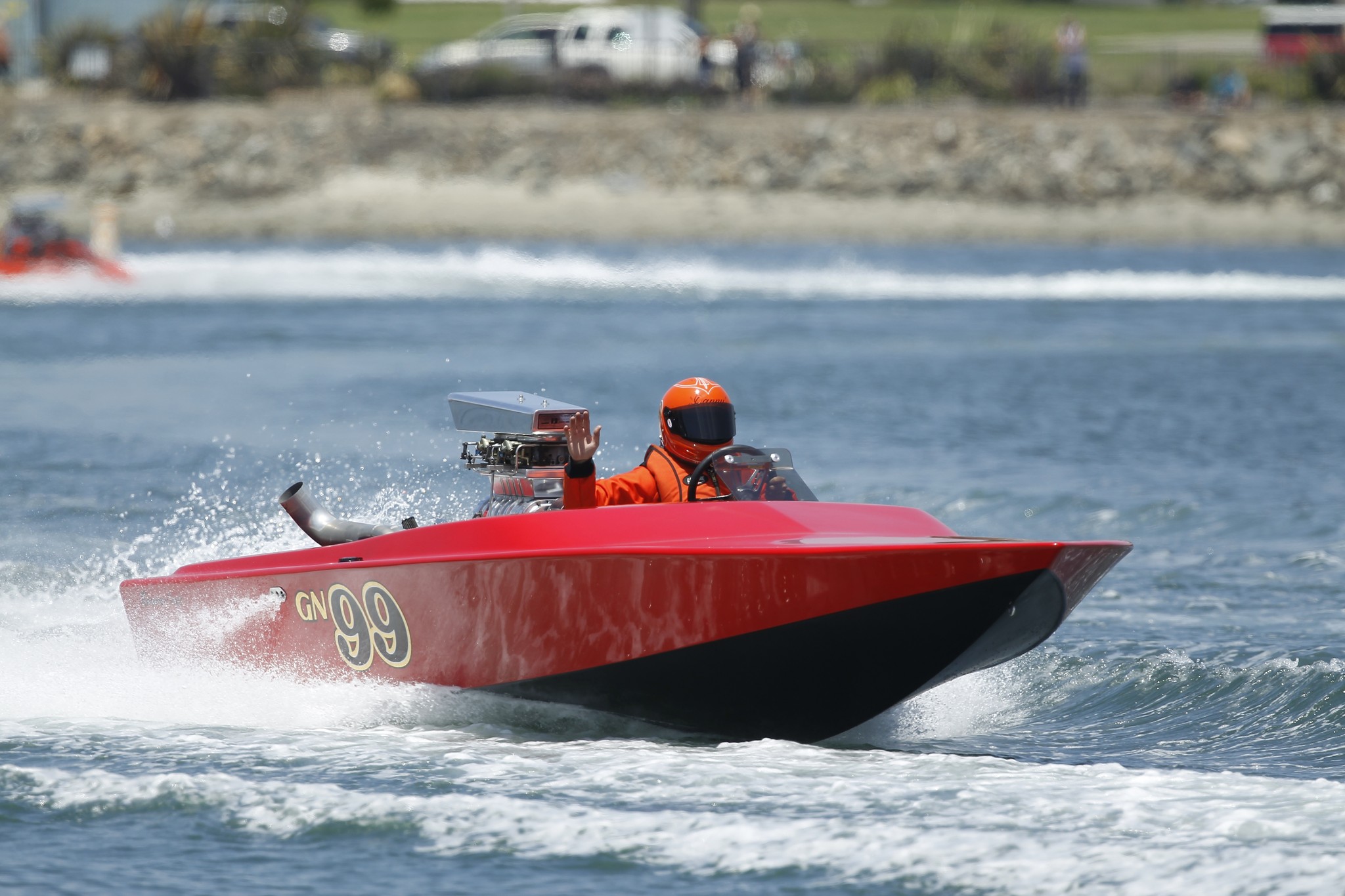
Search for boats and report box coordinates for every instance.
[0,221,131,280]
[119,388,1134,747]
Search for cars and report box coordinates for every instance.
[59,8,395,101]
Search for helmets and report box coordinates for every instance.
[659,377,736,464]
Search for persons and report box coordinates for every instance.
[563,377,796,511]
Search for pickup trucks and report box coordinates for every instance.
[417,11,704,91]
